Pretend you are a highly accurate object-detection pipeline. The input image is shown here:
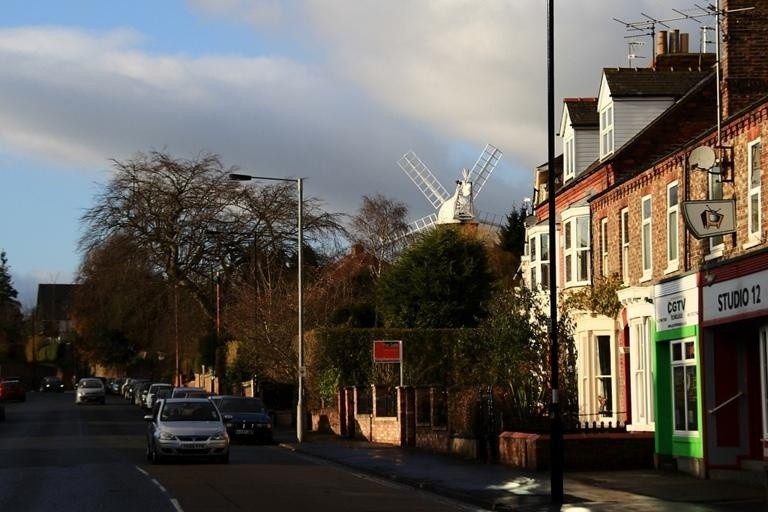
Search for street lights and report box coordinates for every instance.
[176,262,221,393]
[204,230,259,397]
[229,174,306,444]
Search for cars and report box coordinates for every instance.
[76,375,275,465]
[40,376,64,393]
[0,376,26,401]
